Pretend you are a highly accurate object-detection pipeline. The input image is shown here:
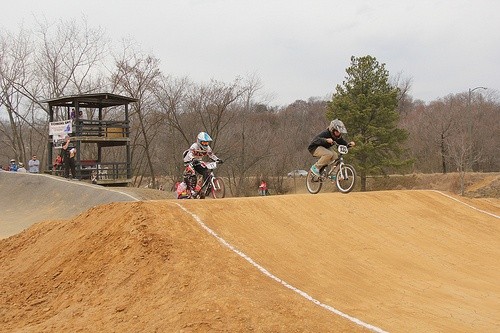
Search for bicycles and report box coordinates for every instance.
[305,141,359,195]
[177,157,225,199]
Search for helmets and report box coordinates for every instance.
[197,131,213,150]
[328,119,347,141]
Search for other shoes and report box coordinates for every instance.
[310,164,321,177]
[72,177,79,181]
[66,178,70,181]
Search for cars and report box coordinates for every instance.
[287,169,308,178]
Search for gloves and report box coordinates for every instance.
[193,159,203,165]
[217,159,224,164]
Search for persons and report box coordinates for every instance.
[8,108,97,182]
[308,119,355,182]
[174,132,222,199]
[259,180,266,195]
